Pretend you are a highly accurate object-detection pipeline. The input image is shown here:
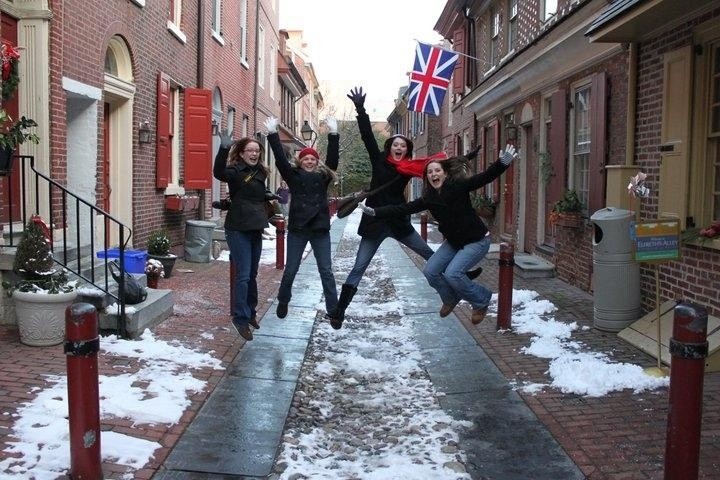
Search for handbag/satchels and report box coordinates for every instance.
[333,184,374,220]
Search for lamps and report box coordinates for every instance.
[211,120,219,136]
[505,120,517,141]
[139,118,152,144]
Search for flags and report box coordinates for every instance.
[405,42,459,117]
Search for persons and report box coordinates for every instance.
[211,127,271,342]
[263,112,344,331]
[356,143,517,326]
[325,85,484,321]
[272,179,292,220]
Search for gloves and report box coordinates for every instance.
[356,200,376,219]
[463,144,484,161]
[346,85,367,110]
[323,114,338,134]
[498,143,519,165]
[261,116,279,133]
[216,127,237,149]
[265,192,282,202]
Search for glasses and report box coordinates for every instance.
[242,148,263,155]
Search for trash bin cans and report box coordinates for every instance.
[183,220,216,263]
[590,207,642,331]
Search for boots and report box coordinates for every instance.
[323,282,358,324]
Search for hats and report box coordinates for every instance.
[384,133,414,153]
[297,146,320,161]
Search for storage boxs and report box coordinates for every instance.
[97,247,148,275]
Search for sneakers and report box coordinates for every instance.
[249,312,260,330]
[329,316,343,330]
[439,290,464,318]
[465,266,483,280]
[230,315,253,342]
[275,296,289,319]
[471,302,489,326]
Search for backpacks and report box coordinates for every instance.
[106,258,149,306]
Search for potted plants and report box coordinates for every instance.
[476,193,495,218]
[145,225,178,279]
[9,218,77,348]
[549,185,587,228]
